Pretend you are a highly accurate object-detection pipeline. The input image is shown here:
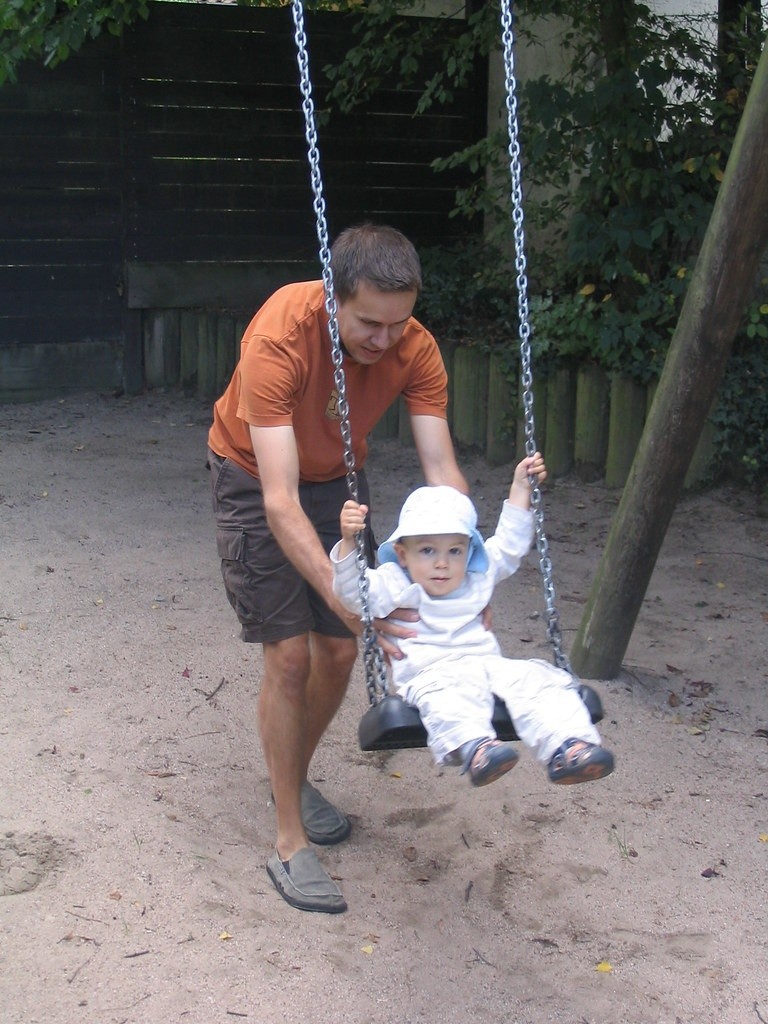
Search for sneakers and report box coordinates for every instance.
[457,735,615,786]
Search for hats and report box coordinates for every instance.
[377,483,490,574]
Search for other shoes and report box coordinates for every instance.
[266,777,351,914]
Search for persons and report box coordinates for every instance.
[329,450,615,787]
[207,217,493,914]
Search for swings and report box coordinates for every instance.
[292,1,606,752]
